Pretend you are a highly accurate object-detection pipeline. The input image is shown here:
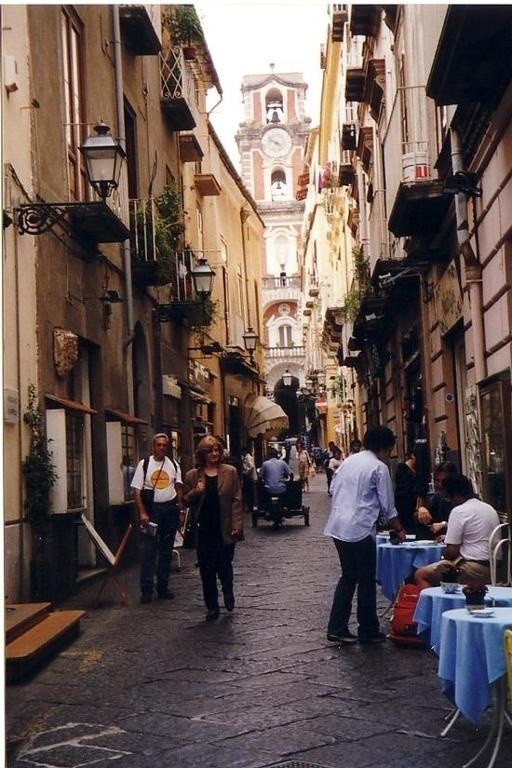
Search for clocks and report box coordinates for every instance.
[261,127,293,158]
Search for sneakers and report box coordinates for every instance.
[157,589,175,600]
[140,593,153,603]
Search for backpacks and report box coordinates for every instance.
[389,582,426,642]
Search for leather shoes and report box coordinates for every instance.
[325,627,358,643]
[224,597,235,612]
[205,606,220,621]
[358,632,386,645]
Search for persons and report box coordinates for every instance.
[179,434,245,622]
[411,472,504,594]
[321,424,409,645]
[239,435,475,535]
[129,431,185,603]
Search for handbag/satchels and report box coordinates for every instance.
[139,488,155,514]
[181,518,201,550]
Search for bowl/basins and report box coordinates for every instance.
[440,583,458,594]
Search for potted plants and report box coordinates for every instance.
[170,5,204,59]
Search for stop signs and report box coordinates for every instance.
[315,398,328,410]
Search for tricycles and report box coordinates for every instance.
[249,480,311,530]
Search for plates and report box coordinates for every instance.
[471,609,495,618]
[414,540,440,546]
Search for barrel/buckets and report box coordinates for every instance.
[402,152,432,188]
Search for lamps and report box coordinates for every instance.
[283,369,297,391]
[152,248,221,322]
[14,115,126,234]
[220,327,259,375]
